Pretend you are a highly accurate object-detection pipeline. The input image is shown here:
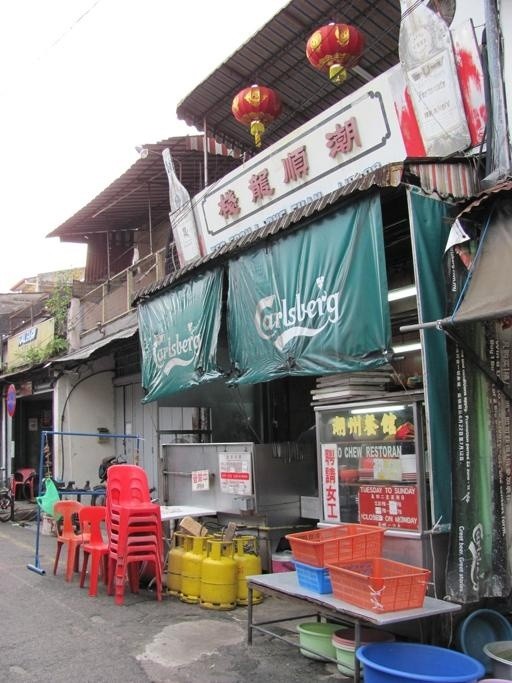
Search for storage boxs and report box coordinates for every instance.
[325,556,432,615]
[284,523,388,569]
[289,559,371,596]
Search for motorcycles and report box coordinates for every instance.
[91,456,127,521]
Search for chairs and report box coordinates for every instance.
[11,468,36,501]
[78,505,111,597]
[104,464,166,607]
[52,499,92,583]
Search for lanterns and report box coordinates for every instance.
[306,20,367,85]
[231,84,282,148]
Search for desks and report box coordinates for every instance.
[244,572,463,683]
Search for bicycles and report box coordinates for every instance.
[24,471,43,503]
[0,467,13,522]
